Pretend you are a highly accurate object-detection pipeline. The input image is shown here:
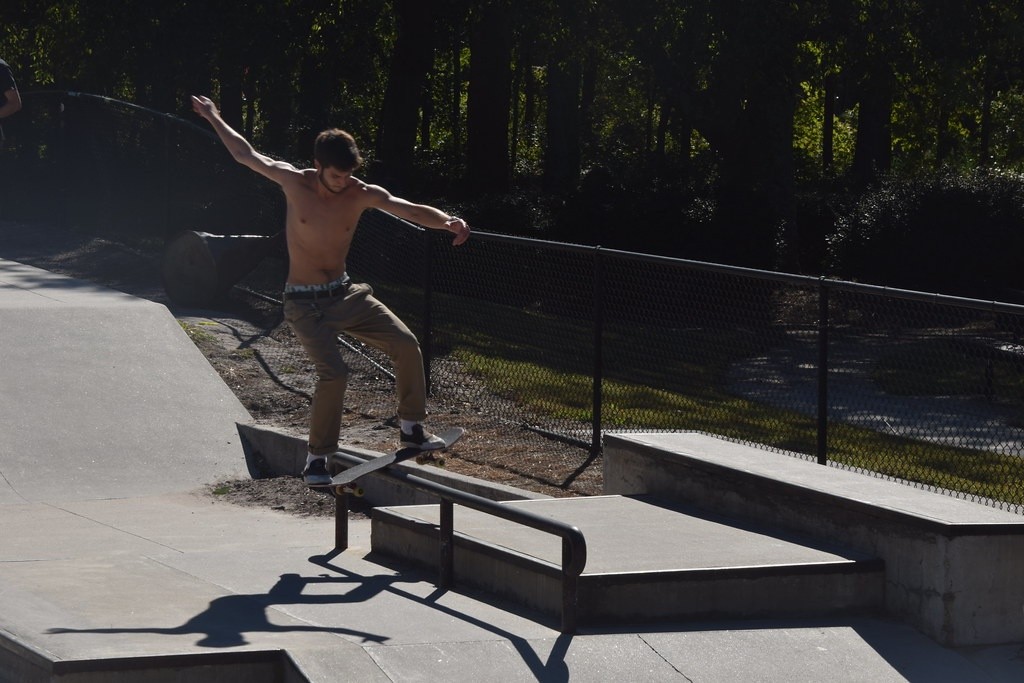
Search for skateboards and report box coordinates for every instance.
[308,426,464,496]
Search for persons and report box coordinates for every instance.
[191,94,471,486]
[0,58,23,119]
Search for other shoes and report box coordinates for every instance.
[400,424,446,449]
[302,458,331,484]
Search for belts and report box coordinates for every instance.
[284,278,352,300]
